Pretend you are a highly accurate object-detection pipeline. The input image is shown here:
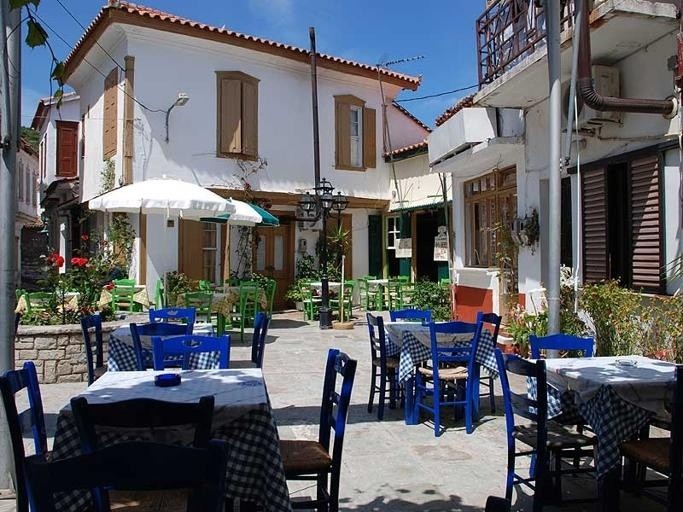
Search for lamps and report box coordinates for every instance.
[164,91,190,144]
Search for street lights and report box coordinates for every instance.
[296,177,350,330]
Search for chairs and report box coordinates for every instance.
[493,348,614,512]
[366,313,406,422]
[454,312,504,415]
[389,311,430,397]
[71,395,227,511]
[2,362,49,512]
[527,333,598,505]
[279,347,358,511]
[130,323,193,370]
[151,334,232,374]
[82,317,109,384]
[618,364,682,510]
[15,277,451,338]
[412,321,482,438]
[228,314,268,370]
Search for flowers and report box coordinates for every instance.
[433,92,475,128]
[40,233,116,324]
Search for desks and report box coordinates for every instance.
[111,323,227,371]
[385,322,499,410]
[527,354,680,508]
[42,366,291,512]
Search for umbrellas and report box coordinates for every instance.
[182,195,280,283]
[87,175,235,308]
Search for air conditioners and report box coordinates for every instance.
[559,62,620,133]
